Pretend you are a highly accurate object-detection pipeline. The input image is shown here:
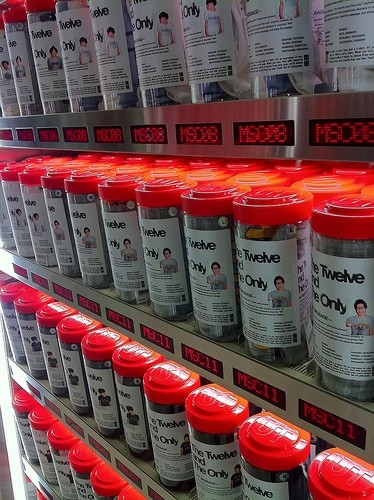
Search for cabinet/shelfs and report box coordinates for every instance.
[0,86,374,500]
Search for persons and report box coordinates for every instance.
[15,207,374,500]
[0,0,300,79]
